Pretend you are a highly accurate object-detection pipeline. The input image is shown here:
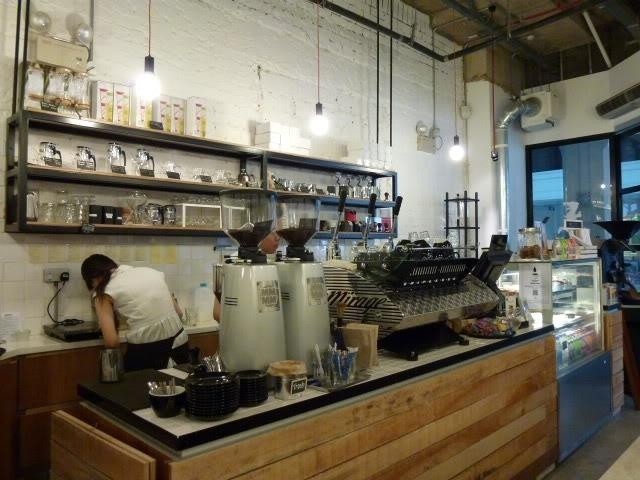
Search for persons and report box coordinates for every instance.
[212,228,280,324]
[81,253,182,372]
[600,233,629,283]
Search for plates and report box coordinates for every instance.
[183,372,241,420]
[234,370,268,406]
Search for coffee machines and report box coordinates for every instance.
[275,193,332,377]
[203,187,277,378]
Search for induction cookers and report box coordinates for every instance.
[43,320,102,341]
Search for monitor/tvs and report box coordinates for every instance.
[472,250,512,280]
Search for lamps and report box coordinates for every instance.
[449,5,467,163]
[136,1,161,100]
[312,2,329,137]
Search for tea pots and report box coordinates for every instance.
[136,146,155,174]
[100,349,120,383]
[104,142,126,173]
[147,204,163,224]
[40,141,62,166]
[74,145,96,170]
[158,161,183,180]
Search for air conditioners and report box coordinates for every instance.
[595,81,640,119]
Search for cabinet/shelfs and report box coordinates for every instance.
[243,148,400,240]
[0,353,22,480]
[21,347,113,479]
[4,111,264,235]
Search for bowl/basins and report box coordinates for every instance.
[147,387,186,419]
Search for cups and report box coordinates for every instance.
[56,189,73,202]
[61,203,76,225]
[161,204,177,225]
[77,203,89,227]
[36,202,55,224]
